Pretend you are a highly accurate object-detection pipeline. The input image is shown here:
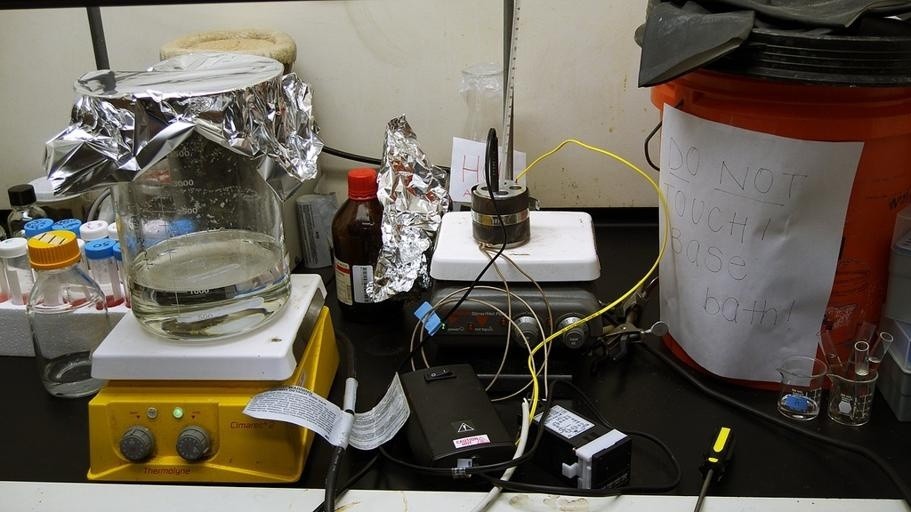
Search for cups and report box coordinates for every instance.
[775,355,828,421]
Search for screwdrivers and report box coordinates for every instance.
[693,423,738,511]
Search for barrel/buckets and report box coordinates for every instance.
[631,0,910,396]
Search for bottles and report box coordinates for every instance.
[26,229,111,399]
[816,321,896,428]
[0,218,199,307]
[7,184,50,238]
[331,166,406,314]
